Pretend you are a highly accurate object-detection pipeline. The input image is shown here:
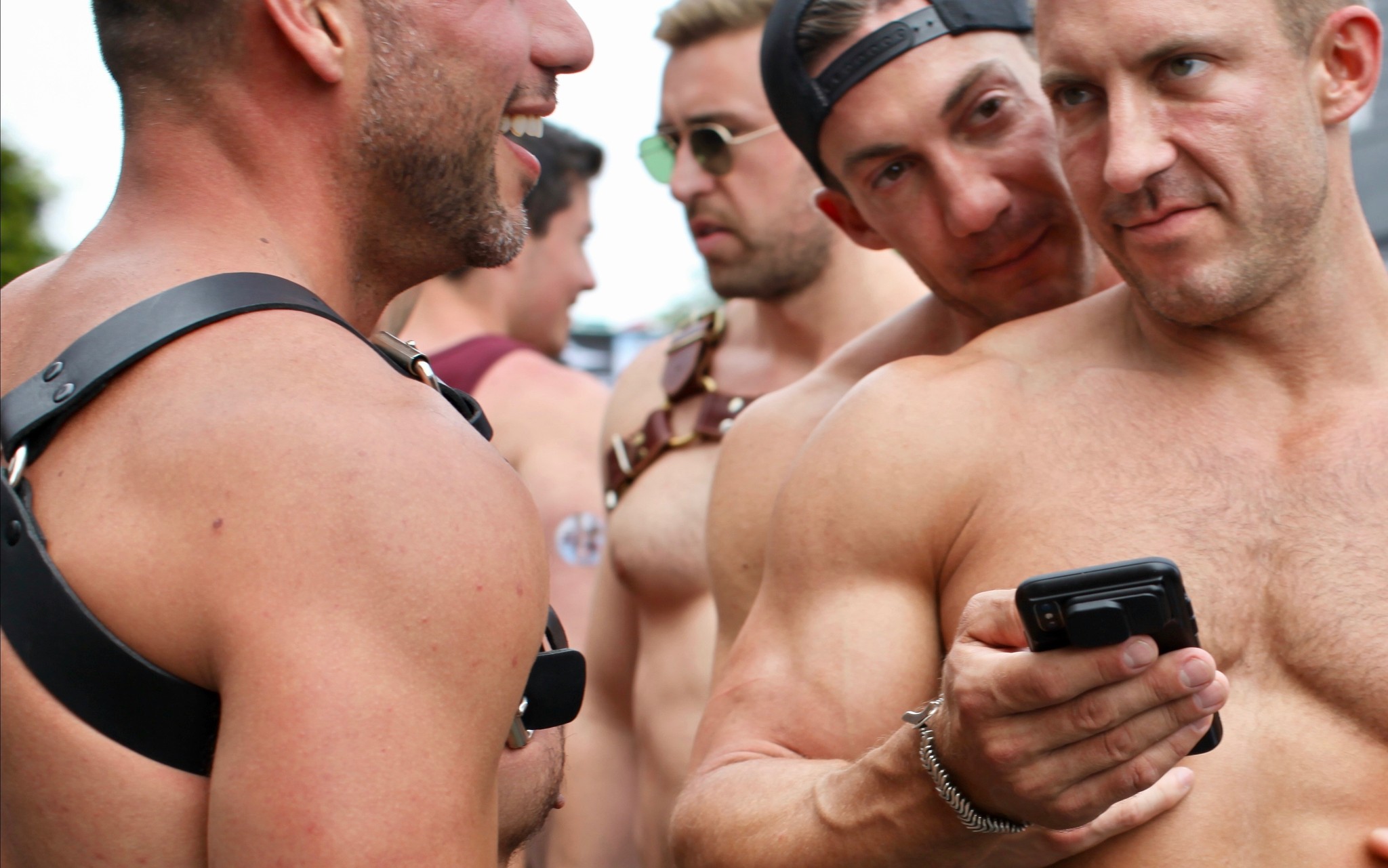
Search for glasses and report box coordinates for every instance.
[638,122,782,184]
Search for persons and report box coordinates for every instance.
[0,0,1386,868]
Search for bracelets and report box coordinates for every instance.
[903,698,1032,834]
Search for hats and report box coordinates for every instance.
[757,0,1037,180]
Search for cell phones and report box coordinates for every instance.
[1015,557,1223,758]
